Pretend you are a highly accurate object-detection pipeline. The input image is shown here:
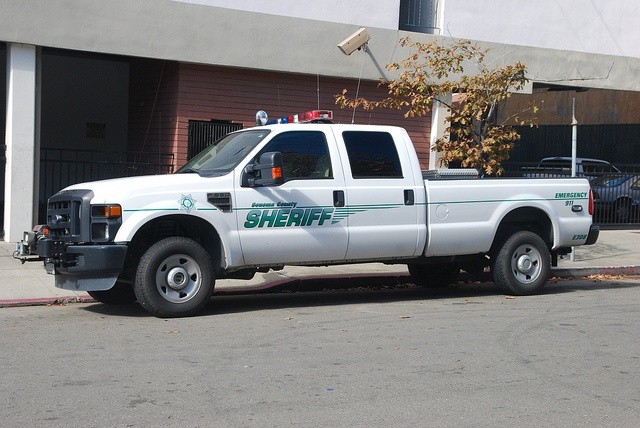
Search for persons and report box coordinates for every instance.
[281,150,310,178]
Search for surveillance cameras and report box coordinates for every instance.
[338,27,370,55]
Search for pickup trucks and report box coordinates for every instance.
[12,109,600,316]
[521,156,627,181]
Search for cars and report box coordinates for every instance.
[588,173,640,224]
